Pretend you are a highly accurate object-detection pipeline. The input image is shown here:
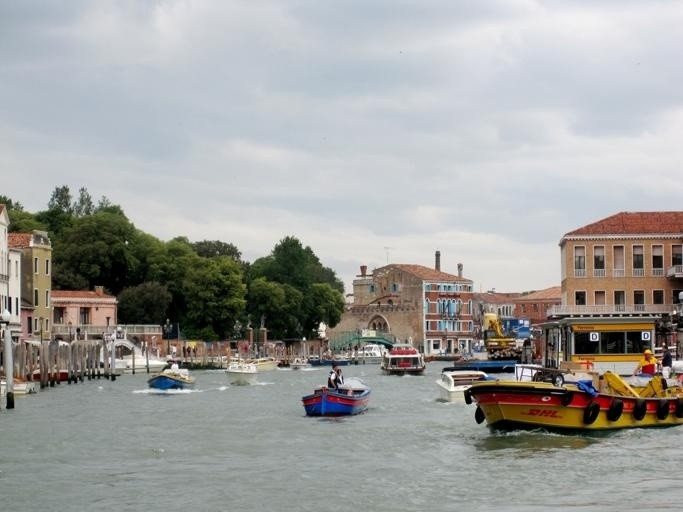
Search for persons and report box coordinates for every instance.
[336,368,344,389]
[660,345,672,379]
[632,349,661,377]
[172,360,183,378]
[162,360,175,372]
[328,364,342,389]
[170,343,199,357]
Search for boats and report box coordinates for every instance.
[290,358,312,370]
[346,344,425,375]
[301,377,370,416]
[225,363,256,386]
[435,371,683,434]
[147,369,194,389]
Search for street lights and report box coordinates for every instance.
[234,320,242,352]
[164,318,173,355]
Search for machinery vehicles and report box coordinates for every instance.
[484,319,516,353]
